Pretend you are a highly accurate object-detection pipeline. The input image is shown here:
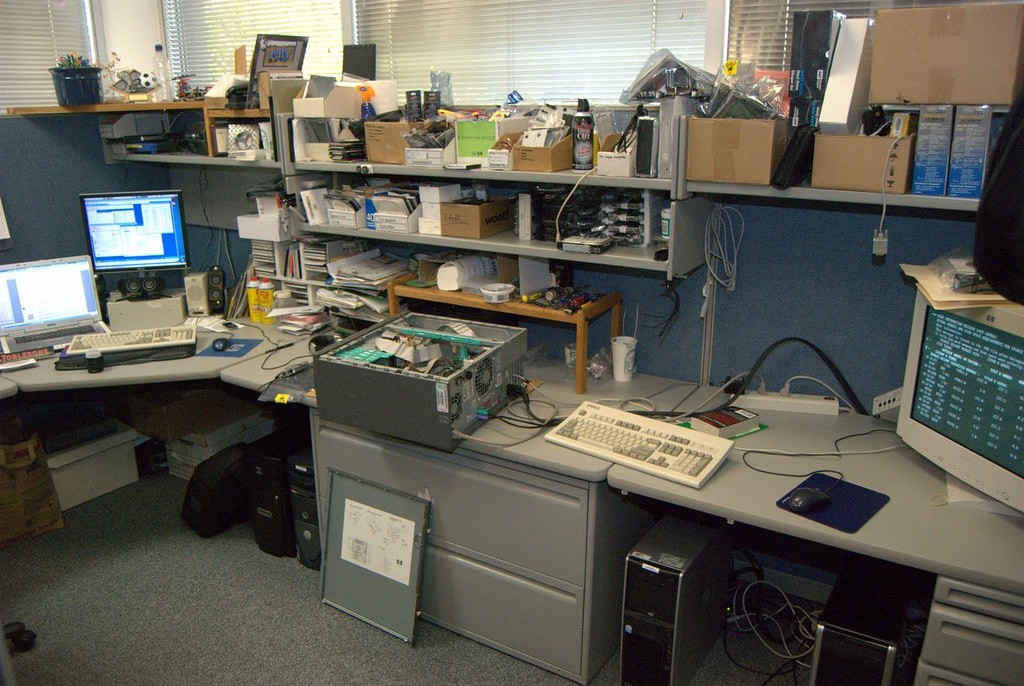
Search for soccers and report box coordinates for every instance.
[139,72,157,89]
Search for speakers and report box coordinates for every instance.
[183,272,209,317]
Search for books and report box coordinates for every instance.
[690,406,760,438]
[252,238,408,334]
[300,185,420,216]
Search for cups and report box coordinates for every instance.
[86,349,104,373]
[565,344,577,367]
[258,122,274,161]
[660,208,671,239]
[611,336,637,382]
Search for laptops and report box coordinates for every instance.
[0,254,111,364]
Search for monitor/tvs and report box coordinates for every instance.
[78,188,191,303]
[897,286,1023,518]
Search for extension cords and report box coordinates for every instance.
[730,389,839,416]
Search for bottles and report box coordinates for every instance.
[246,276,262,323]
[258,277,275,324]
[153,44,173,103]
[572,99,594,172]
[272,290,297,332]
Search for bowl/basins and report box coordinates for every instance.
[481,283,515,303]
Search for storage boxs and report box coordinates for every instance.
[132,379,261,443]
[294,3,1024,239]
[40,417,140,513]
[164,415,277,480]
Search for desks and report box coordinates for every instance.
[0,318,1024,686]
[386,270,623,395]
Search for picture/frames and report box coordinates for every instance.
[244,34,309,110]
[228,123,260,157]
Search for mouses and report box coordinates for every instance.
[788,487,830,513]
[212,337,230,352]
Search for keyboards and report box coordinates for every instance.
[542,400,736,489]
[55,324,198,371]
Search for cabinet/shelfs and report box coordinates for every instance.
[7,98,978,328]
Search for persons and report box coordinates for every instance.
[329,141,365,163]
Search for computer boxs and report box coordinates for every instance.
[809,553,915,686]
[314,309,529,453]
[245,423,321,572]
[619,512,738,686]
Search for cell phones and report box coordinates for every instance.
[223,322,238,330]
